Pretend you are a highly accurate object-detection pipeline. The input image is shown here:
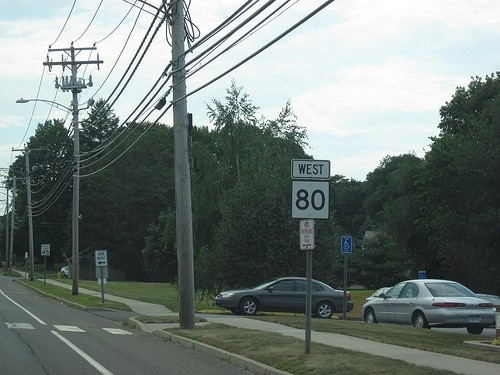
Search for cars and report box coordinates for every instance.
[213,277,355,321]
[366,287,392,302]
[360,279,497,332]
[61,265,71,277]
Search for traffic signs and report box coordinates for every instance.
[95,250,107,267]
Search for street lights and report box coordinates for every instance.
[16,98,80,297]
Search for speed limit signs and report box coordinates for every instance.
[291,180,330,221]
[41,244,50,256]
